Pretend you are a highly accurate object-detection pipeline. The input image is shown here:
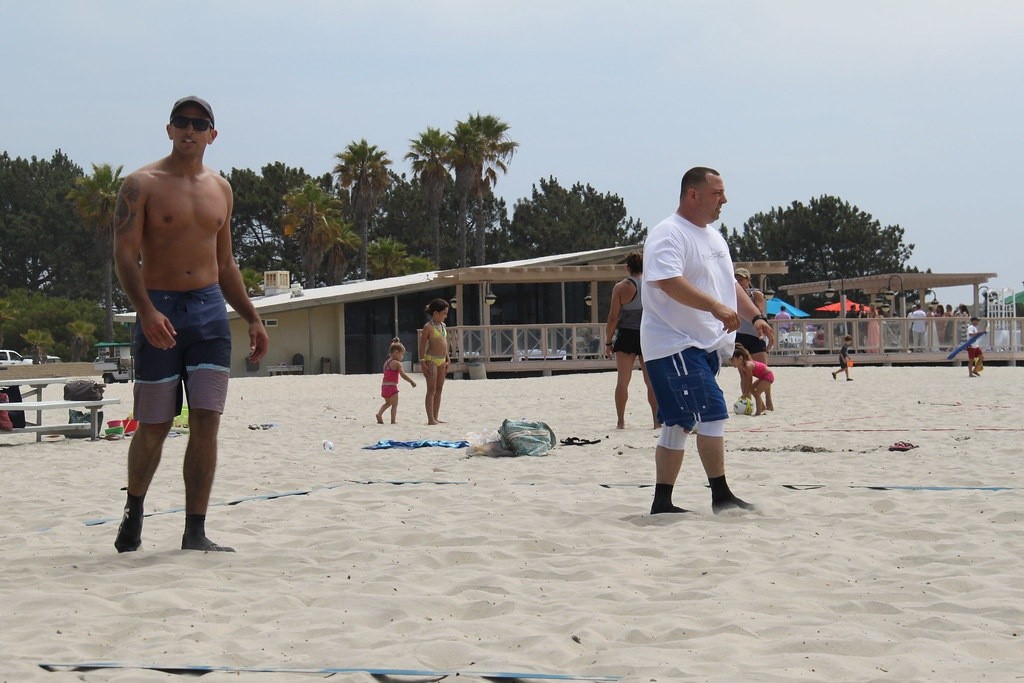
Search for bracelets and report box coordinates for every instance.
[419,359,425,364]
[606,342,612,346]
[710,301,719,312]
[751,313,769,325]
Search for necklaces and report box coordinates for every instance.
[430,318,447,337]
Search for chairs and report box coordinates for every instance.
[761,330,818,356]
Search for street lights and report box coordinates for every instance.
[884,274,908,353]
[824,270,848,339]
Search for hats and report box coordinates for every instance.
[734,267,750,278]
[171,96,215,127]
[971,317,981,322]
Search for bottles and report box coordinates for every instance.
[322,440,333,450]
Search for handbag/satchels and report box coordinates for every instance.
[0,393,13,430]
[64,409,103,438]
[974,356,984,372]
[1,384,27,429]
[63,378,103,401]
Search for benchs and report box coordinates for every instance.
[0,399,121,442]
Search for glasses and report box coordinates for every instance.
[170,114,215,132]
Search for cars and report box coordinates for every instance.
[0,350,42,368]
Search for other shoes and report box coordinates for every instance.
[650,502,690,514]
[181,535,234,552]
[711,499,757,515]
[115,504,144,553]
[846,379,853,381]
[832,372,836,379]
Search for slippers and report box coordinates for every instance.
[561,436,602,446]
[249,423,271,430]
[889,441,919,451]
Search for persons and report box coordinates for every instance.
[114,96,268,556]
[640,167,775,515]
[907,304,972,353]
[846,304,884,354]
[832,334,853,381]
[419,299,450,425]
[733,268,774,411]
[375,337,416,425]
[775,306,791,355]
[605,250,664,432]
[967,317,985,377]
[729,342,774,415]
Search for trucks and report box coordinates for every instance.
[100,356,135,384]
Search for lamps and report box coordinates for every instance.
[485,280,497,305]
[583,295,591,306]
[449,293,456,309]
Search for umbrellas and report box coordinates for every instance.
[764,297,810,317]
[815,299,879,312]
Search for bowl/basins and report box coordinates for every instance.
[105,427,123,435]
[107,421,121,428]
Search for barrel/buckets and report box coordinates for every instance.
[847,361,854,367]
[122,420,138,433]
[172,405,190,433]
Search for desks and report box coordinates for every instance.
[0,376,106,437]
